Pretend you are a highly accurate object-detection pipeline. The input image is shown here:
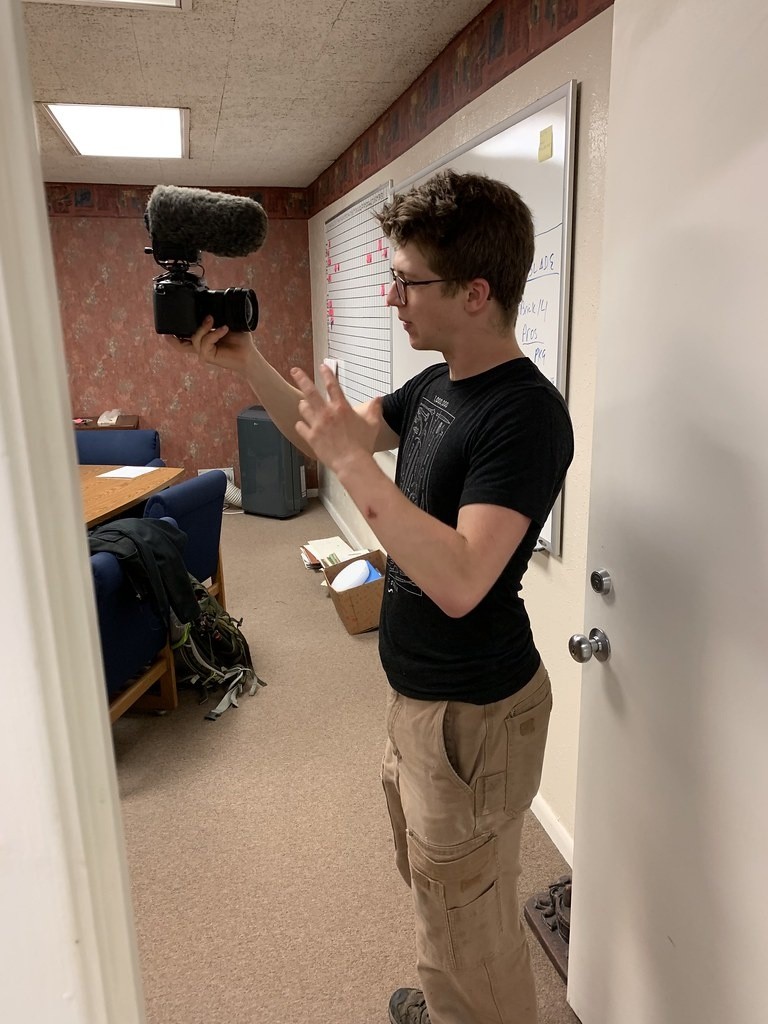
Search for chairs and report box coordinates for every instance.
[72,425,167,467]
[145,471,232,635]
[90,514,181,742]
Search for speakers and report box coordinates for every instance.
[236,404,308,517]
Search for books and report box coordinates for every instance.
[299,535,371,570]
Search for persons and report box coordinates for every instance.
[162,169,577,1024]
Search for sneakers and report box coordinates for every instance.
[388,986,431,1024]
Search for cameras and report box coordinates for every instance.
[152,272,258,339]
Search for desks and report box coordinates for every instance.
[77,459,198,528]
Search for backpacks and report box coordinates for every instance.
[174,568,267,721]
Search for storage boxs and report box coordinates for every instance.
[324,549,392,636]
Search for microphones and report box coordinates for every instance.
[148,187,267,262]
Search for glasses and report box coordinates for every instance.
[389,267,491,307]
